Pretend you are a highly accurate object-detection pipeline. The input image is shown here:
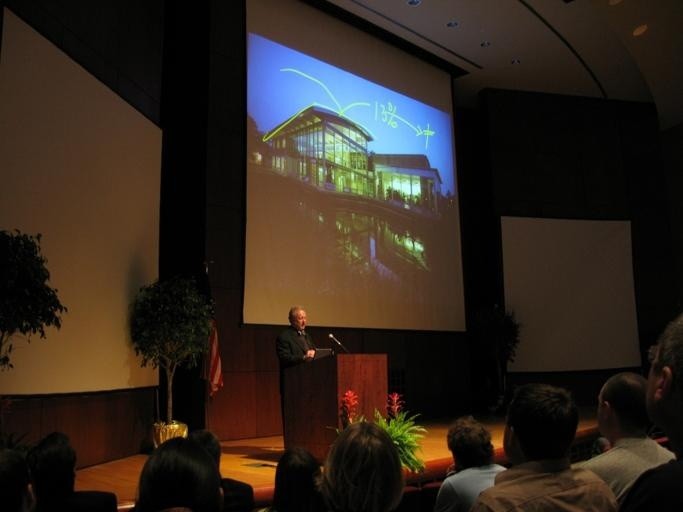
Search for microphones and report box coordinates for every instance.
[328,334,341,345]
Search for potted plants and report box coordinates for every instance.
[129,271,214,451]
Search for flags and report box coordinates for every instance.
[202,272,223,400]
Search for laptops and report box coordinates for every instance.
[313,348,334,361]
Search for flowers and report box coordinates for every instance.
[336,388,428,471]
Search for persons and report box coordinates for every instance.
[134,431,321,511]
[434,417,506,511]
[313,421,404,511]
[1,431,101,511]
[274,304,320,461]
[470,315,682,512]
